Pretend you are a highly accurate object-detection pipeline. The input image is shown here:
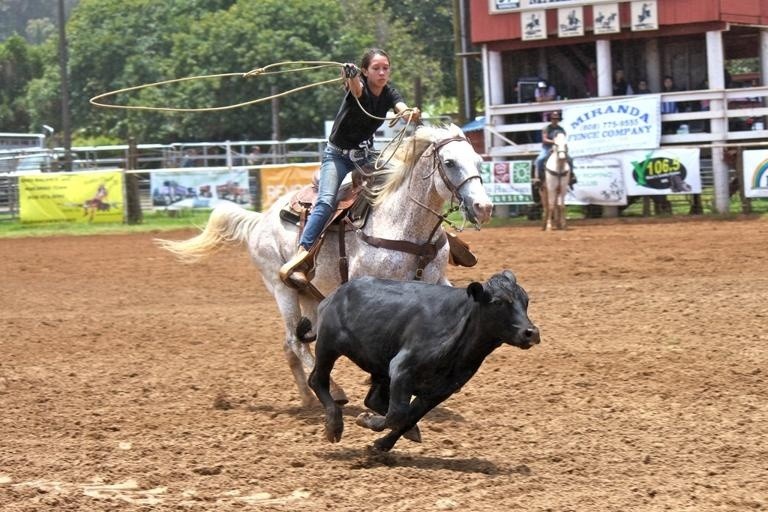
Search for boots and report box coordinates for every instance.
[286,244,312,285]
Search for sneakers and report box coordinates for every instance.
[571,177,577,183]
[534,178,541,190]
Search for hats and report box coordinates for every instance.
[537,80,549,88]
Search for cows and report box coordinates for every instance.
[295,270,541,456]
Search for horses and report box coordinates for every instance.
[151,123,496,413]
[534,131,572,233]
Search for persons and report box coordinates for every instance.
[532,110,577,183]
[181,149,197,167]
[583,62,763,134]
[288,50,421,289]
[533,81,556,101]
[247,145,260,165]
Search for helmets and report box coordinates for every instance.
[550,111,560,119]
